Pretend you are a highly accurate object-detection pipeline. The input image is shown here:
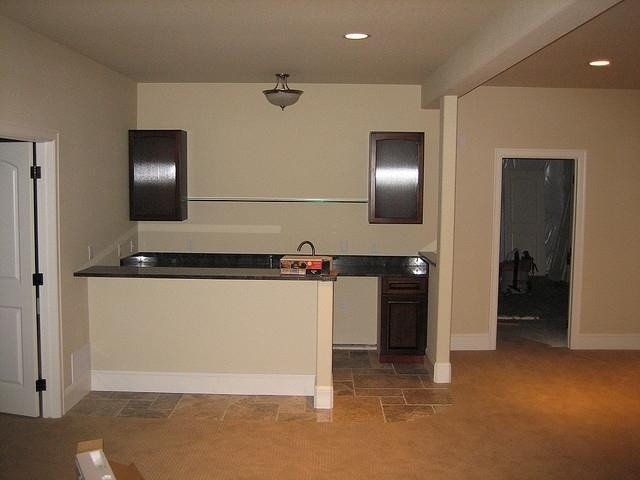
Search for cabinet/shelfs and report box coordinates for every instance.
[368,131,425,224]
[128,128,189,222]
[378,277,428,355]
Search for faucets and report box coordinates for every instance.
[296,239,316,256]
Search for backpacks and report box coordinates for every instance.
[499,248,533,297]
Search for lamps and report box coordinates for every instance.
[262,72,305,112]
